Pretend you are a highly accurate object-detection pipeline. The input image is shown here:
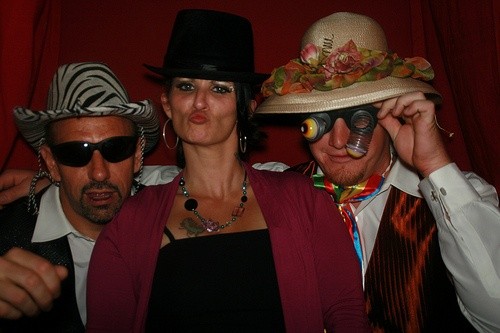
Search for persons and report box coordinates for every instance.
[0,9,500,333]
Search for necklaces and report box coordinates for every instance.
[180,163,249,237]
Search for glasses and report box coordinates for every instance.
[45,135,138,167]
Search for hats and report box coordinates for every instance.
[255,12,442,113]
[13,63,160,155]
[143,9,272,85]
